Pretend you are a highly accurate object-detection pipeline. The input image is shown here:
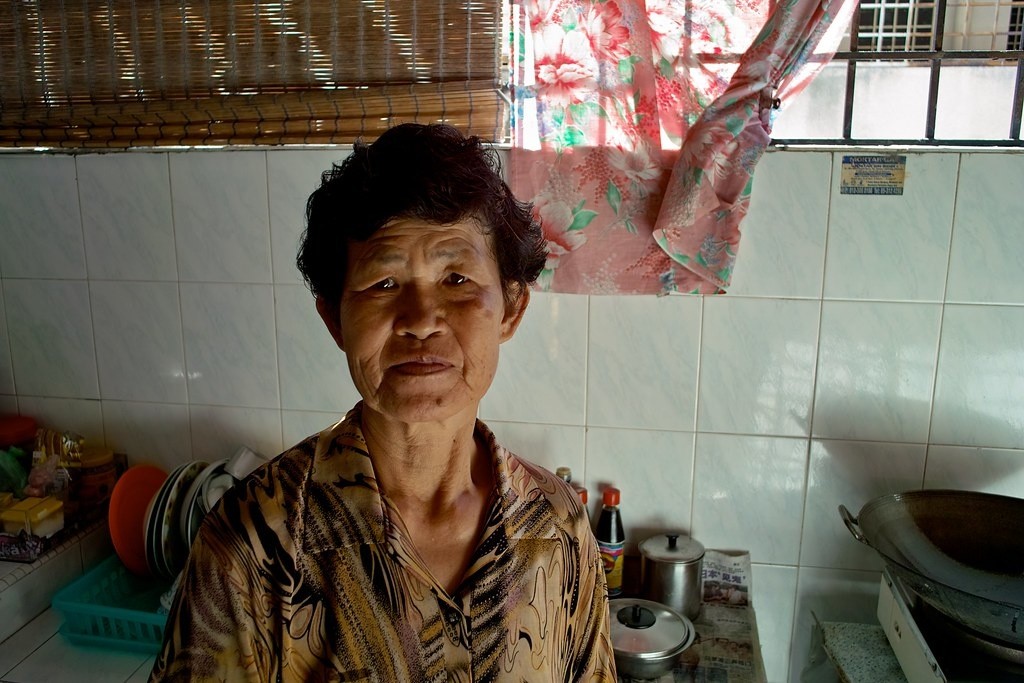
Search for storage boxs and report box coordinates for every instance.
[0,496,65,538]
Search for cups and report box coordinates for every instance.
[637,533,705,620]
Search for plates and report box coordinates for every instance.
[109,458,230,577]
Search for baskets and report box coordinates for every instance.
[51,555,170,654]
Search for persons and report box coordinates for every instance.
[149,122,617,683]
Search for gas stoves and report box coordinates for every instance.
[877,565,1024,683]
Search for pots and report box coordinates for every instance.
[838,489,1024,645]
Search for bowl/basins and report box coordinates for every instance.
[609,599,696,678]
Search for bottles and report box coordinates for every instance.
[596,488,626,599]
[556,467,571,484]
[575,487,591,531]
